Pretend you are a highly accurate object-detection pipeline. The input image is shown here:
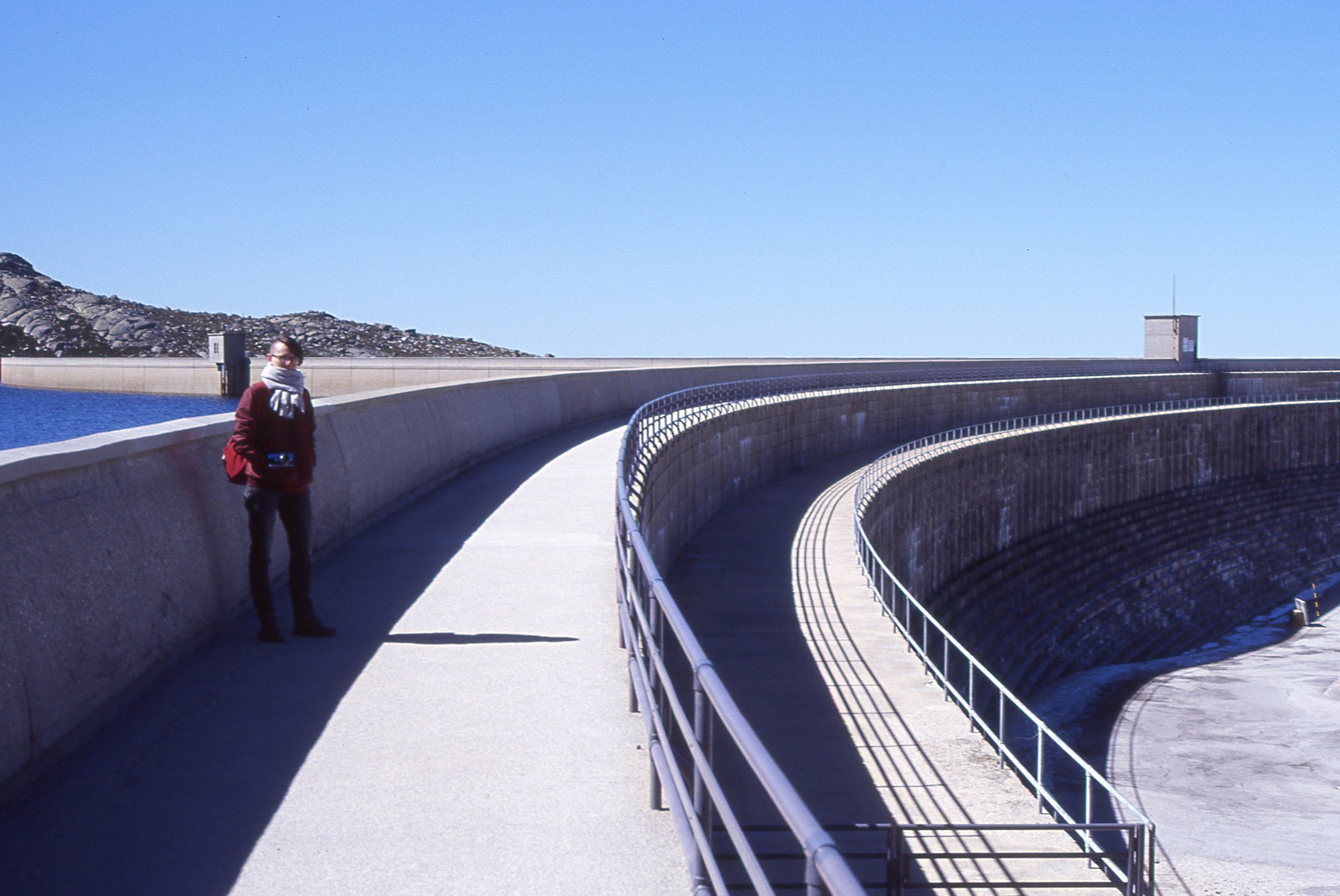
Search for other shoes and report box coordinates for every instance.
[257,617,283,643]
[295,610,338,638]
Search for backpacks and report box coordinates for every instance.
[222,380,269,483]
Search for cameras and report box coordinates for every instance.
[267,453,295,469]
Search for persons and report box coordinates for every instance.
[230,337,337,644]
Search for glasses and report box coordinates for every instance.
[269,354,304,367]
